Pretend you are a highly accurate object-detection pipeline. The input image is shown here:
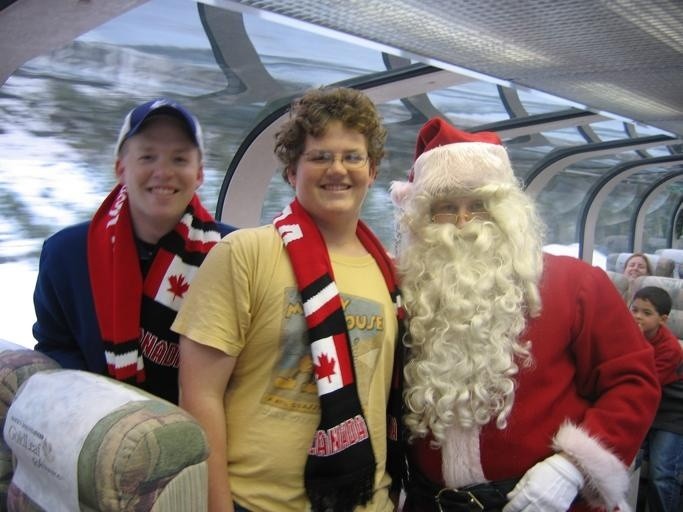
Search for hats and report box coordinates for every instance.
[388,116,512,208]
[116,97,204,161]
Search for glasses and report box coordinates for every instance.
[301,149,371,170]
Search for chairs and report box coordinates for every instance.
[602,247,683,343]
[0,341,210,510]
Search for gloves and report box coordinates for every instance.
[501,453,586,512]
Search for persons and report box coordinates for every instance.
[387,117,662,512]
[623,253,652,279]
[169,85,407,512]
[31,99,242,407]
[627,286,683,512]
